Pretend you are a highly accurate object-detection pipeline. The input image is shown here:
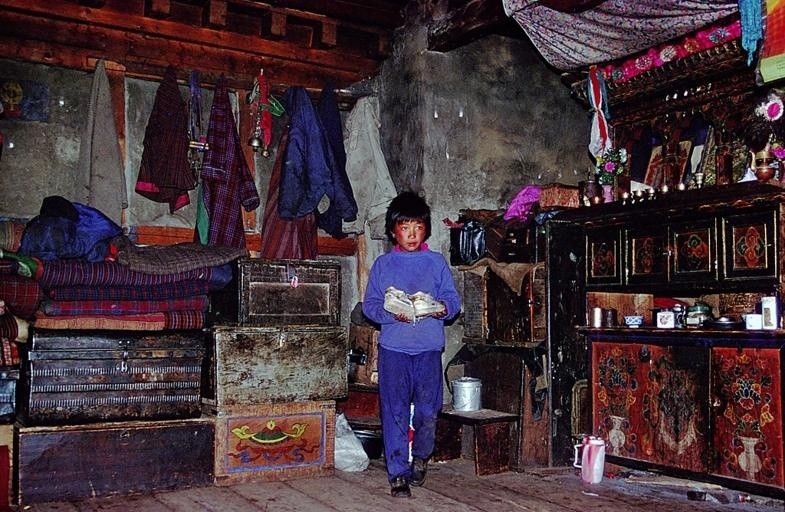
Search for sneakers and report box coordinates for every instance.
[383,286,445,322]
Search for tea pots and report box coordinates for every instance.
[749,134,776,183]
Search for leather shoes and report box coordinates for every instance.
[391,456,428,498]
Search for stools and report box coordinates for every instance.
[431,403,519,476]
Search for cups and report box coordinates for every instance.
[571,437,606,486]
[657,311,675,329]
[591,307,602,328]
[761,297,777,331]
[745,314,763,330]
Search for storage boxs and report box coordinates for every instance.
[13,419,215,505]
[202,398,336,489]
[450,182,581,468]
[216,257,342,325]
[347,322,381,385]
[333,383,383,424]
[206,323,350,406]
[1,420,16,508]
[25,333,210,420]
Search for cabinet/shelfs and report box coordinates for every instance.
[560,1,784,500]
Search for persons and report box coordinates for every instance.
[363,193,461,499]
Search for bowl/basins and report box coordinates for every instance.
[714,320,737,330]
[624,315,644,328]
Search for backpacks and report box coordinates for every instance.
[448,218,487,266]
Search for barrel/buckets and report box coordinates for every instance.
[580,435,605,484]
[451,378,483,410]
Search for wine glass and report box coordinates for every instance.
[694,172,705,190]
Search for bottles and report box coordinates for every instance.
[684,305,714,328]
